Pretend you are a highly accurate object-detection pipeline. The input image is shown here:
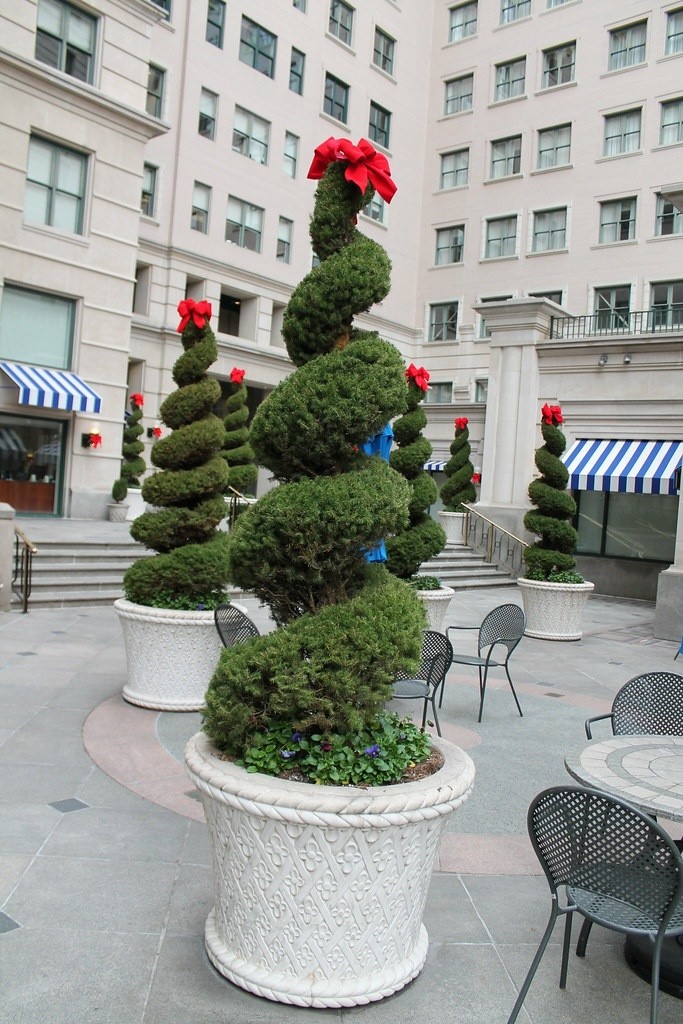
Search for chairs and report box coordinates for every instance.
[585,672,683,737]
[215,604,261,648]
[439,604,526,724]
[507,785,683,1024]
[382,631,453,737]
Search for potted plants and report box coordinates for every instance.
[388,366,454,635]
[516,404,594,640]
[115,298,249,711]
[437,417,477,544]
[122,393,148,520]
[187,136,475,1010]
[220,367,258,533]
[108,479,128,521]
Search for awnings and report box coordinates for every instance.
[0,428,27,461]
[1,361,102,413]
[557,439,683,497]
[421,459,451,473]
[33,437,58,465]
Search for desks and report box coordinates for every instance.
[564,735,682,827]
